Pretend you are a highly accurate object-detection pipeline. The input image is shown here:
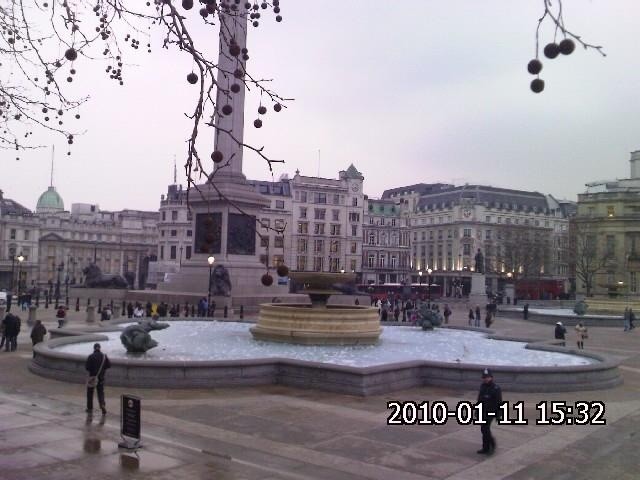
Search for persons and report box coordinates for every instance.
[621,305,631,332]
[83,342,111,415]
[4,281,60,314]
[432,303,436,309]
[370,294,426,323]
[471,367,503,456]
[126,301,133,319]
[55,305,65,329]
[574,320,589,349]
[150,303,158,317]
[156,301,167,317]
[0,313,12,348]
[468,308,474,327]
[145,301,153,316]
[474,304,481,328]
[626,308,636,330]
[201,296,208,317]
[209,301,216,318]
[197,299,203,317]
[354,297,360,305]
[132,303,145,319]
[443,303,453,324]
[104,306,113,320]
[168,304,177,317]
[183,301,191,317]
[474,247,486,275]
[100,307,108,321]
[554,321,567,347]
[1,312,21,352]
[434,305,440,312]
[484,312,494,328]
[29,319,47,359]
[522,303,530,320]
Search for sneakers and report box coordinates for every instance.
[101,404,107,415]
[86,409,92,413]
[477,448,489,453]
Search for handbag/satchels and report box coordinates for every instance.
[583,330,588,338]
[85,376,99,388]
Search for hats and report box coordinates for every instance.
[481,368,493,378]
[556,322,562,326]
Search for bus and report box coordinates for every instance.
[514,278,565,301]
[354,282,442,304]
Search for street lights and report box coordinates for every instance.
[7,254,27,313]
[418,268,434,309]
[205,254,215,317]
